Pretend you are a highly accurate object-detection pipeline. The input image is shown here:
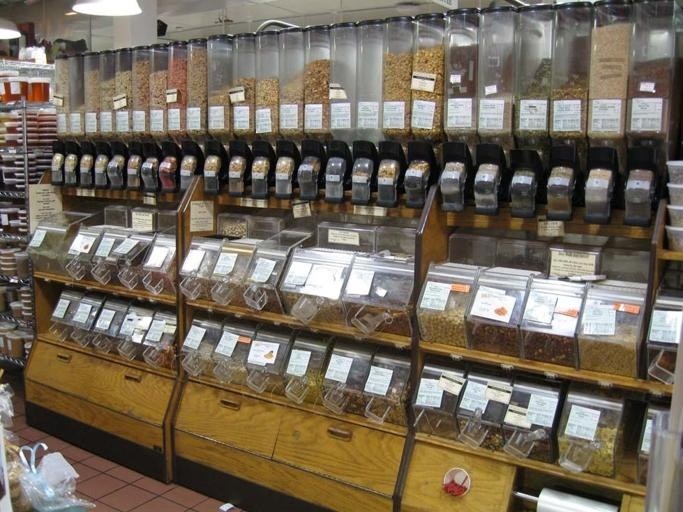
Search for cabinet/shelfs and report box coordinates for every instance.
[0,95,58,367]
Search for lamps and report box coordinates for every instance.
[70,0,142,18]
[0,17,22,41]
